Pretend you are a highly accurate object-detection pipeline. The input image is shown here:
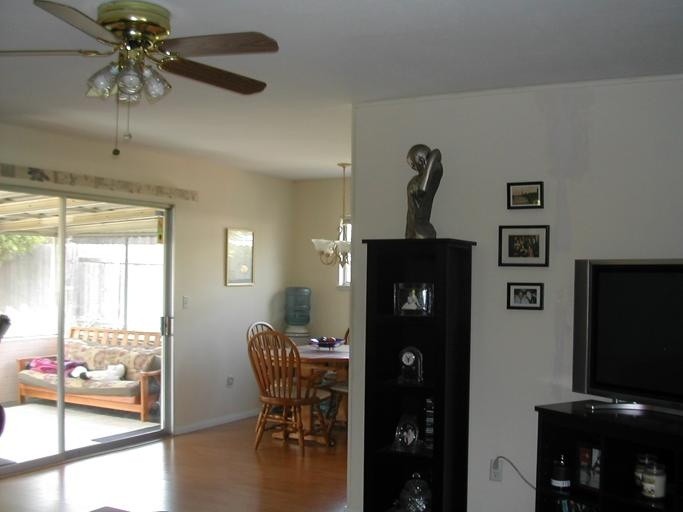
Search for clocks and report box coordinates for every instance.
[394,345,425,386]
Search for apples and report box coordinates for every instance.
[318,336,337,343]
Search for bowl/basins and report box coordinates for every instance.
[306,337,345,348]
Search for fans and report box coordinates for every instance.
[0,0,280,96]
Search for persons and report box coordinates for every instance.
[514,289,526,304]
[509,235,539,258]
[523,291,536,304]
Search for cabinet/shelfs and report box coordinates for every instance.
[358,236,480,512]
[531,397,682,512]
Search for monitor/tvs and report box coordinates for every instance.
[572,258,683,416]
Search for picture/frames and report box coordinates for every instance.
[222,226,256,288]
[496,223,551,268]
[505,281,544,311]
[505,180,544,209]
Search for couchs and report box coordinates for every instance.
[15,325,162,423]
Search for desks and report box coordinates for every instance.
[259,342,349,449]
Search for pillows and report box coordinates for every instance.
[62,338,162,382]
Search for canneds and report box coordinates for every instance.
[551,453,571,493]
[635,455,667,502]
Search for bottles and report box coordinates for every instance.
[549,453,572,489]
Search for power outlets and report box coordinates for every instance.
[487,457,503,484]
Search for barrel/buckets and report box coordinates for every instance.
[284,287,312,326]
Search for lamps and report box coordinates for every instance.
[309,162,351,268]
[85,2,173,160]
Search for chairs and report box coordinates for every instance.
[326,326,350,438]
[247,331,333,464]
[246,321,334,435]
